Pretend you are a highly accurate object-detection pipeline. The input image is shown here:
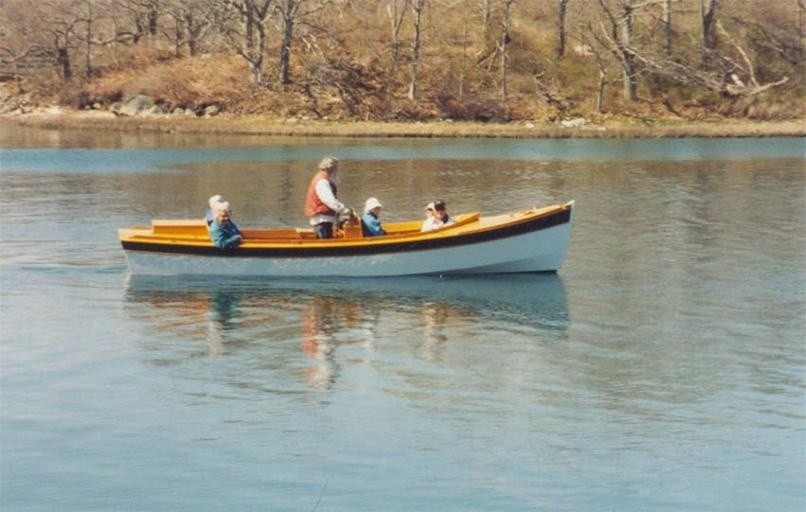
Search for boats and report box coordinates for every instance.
[117,198,576,278]
[107,270,576,351]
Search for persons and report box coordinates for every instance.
[360,197,386,237]
[206,195,243,253]
[421,201,456,232]
[304,156,352,238]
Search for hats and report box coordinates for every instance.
[364,196,382,214]
[424,200,446,212]
